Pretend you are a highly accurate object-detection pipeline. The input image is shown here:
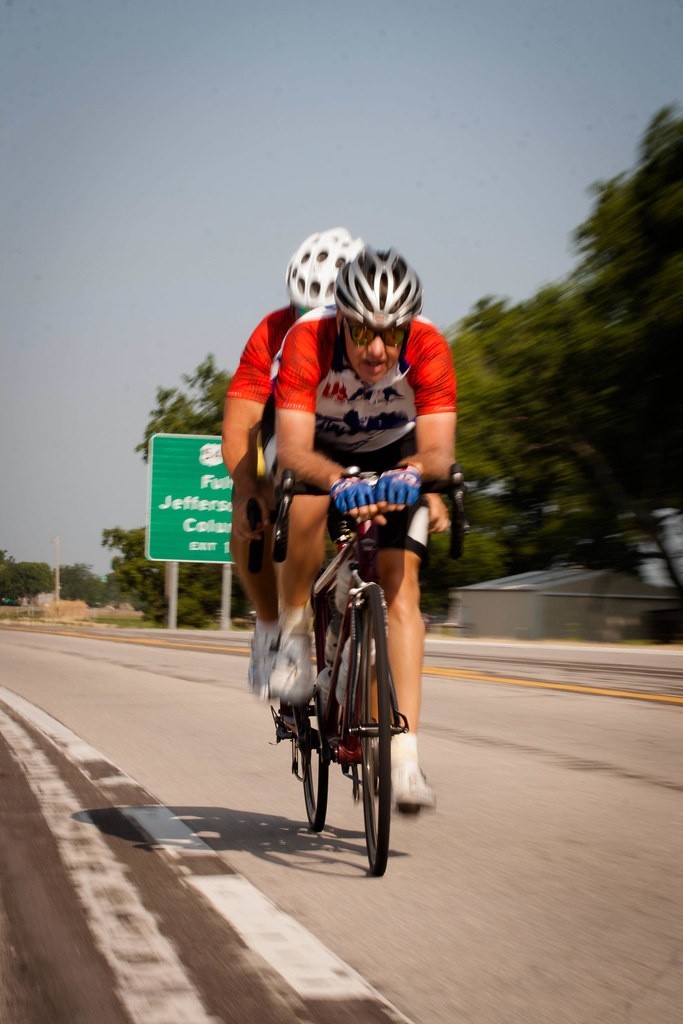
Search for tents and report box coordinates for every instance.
[450,569,678,644]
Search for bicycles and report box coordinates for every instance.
[243,461,470,879]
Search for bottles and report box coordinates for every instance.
[315,667,334,712]
[324,622,342,669]
[334,633,378,709]
[334,561,360,614]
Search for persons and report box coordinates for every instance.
[222,228,375,705]
[268,249,456,809]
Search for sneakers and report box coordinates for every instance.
[270,631,314,705]
[246,623,282,699]
[389,764,436,809]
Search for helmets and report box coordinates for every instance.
[333,245,423,330]
[285,227,365,311]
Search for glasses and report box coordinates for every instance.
[343,316,406,347]
